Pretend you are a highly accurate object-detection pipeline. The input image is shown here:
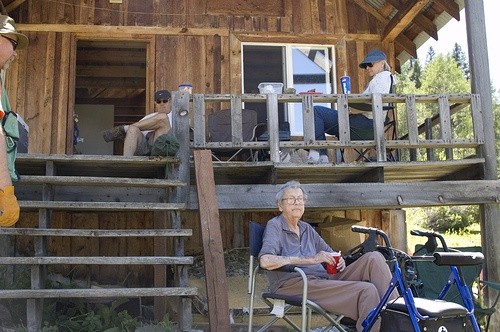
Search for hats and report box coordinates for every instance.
[154,90,171,101]
[0,15,29,49]
[359,49,387,69]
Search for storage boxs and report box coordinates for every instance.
[258,82,283,94]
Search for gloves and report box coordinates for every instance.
[0,185,19,227]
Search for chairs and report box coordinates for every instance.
[340,74,401,162]
[209,109,290,162]
[247,221,346,332]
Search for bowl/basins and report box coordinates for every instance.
[178,84,193,94]
[257,82,284,95]
[284,88,297,94]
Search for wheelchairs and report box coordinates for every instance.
[343,224,452,298]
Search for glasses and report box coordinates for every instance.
[1,34,18,51]
[156,99,169,104]
[363,62,373,69]
[281,196,304,205]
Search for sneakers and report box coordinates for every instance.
[103,125,126,142]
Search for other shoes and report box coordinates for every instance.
[309,155,328,163]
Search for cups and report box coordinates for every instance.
[325,252,341,274]
[339,76,351,95]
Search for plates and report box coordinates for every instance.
[298,92,324,94]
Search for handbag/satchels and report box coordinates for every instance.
[3,110,30,154]
[151,133,181,157]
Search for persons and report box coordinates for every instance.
[312,50,390,164]
[0,13,29,227]
[103,90,191,157]
[258,181,399,332]
